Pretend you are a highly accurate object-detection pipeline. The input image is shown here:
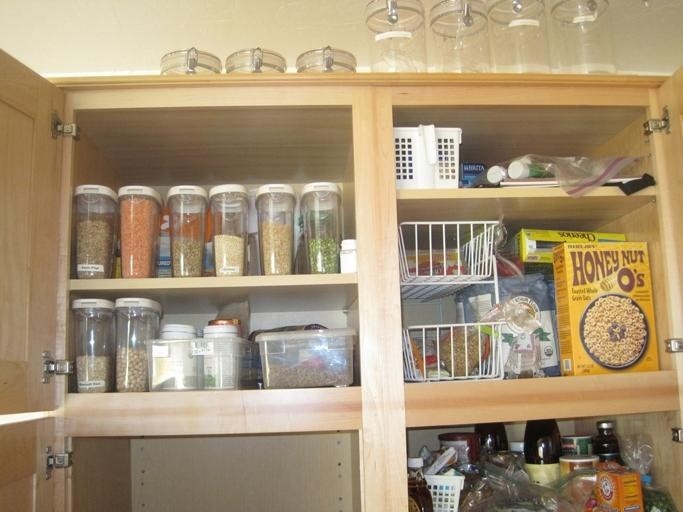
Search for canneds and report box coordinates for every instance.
[437,432,475,462]
[558,453,598,477]
[561,433,595,455]
[209,318,242,338]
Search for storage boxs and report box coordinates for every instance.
[255,328,356,388]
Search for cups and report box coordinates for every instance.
[523,461,560,501]
[158,46,358,79]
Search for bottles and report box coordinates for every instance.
[595,420,621,464]
[407,457,434,511]
[72,181,342,280]
[71,296,162,392]
[365,0,618,76]
[338,237,356,275]
[160,323,240,392]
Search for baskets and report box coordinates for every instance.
[403,321,503,382]
[398,221,499,300]
[394,124,462,189]
[423,467,465,512]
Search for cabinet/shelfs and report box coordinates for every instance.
[1,50,682,511]
